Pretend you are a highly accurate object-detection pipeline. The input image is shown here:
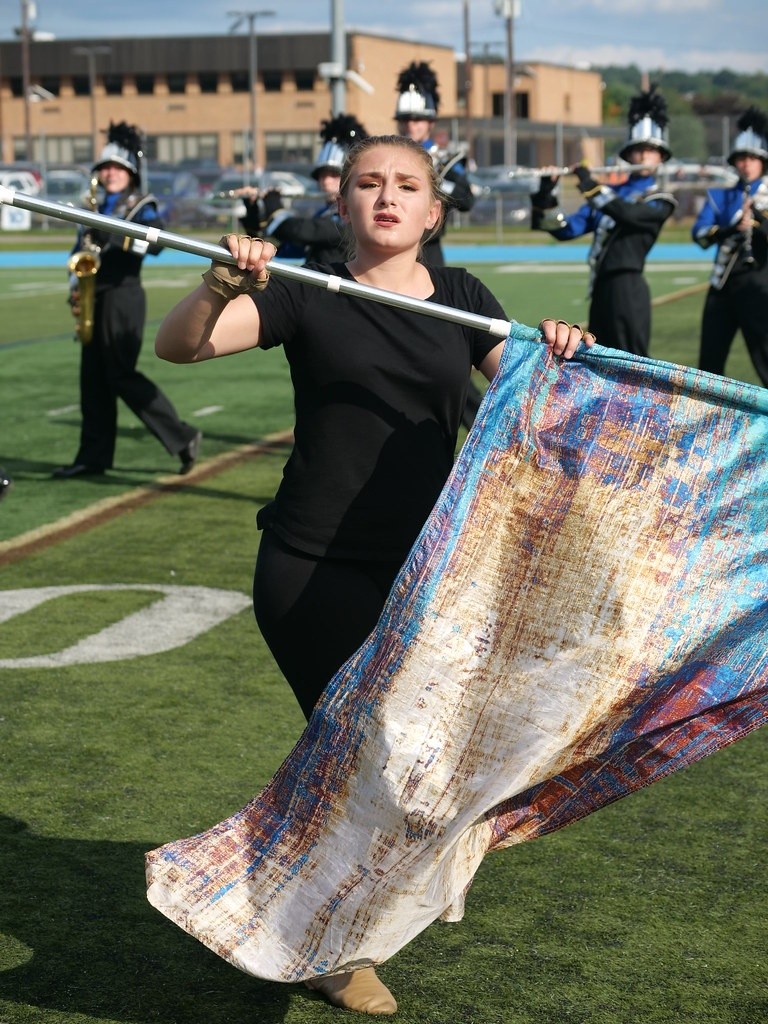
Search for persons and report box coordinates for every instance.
[50,143,202,479]
[689,127,768,390]
[522,116,678,360]
[238,140,348,260]
[153,133,599,1018]
[391,85,487,431]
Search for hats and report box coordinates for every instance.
[394,62,440,119]
[728,107,768,176]
[619,84,674,166]
[310,114,371,181]
[92,120,141,188]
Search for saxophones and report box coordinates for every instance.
[66,177,103,340]
[710,185,756,290]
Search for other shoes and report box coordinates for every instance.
[53,466,104,480]
[304,966,397,1015]
[179,432,202,474]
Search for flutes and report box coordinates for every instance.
[214,190,341,199]
[508,163,658,179]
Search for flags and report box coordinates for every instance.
[146,320,768,984]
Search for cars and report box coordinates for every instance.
[657,163,740,223]
[0,163,560,235]
